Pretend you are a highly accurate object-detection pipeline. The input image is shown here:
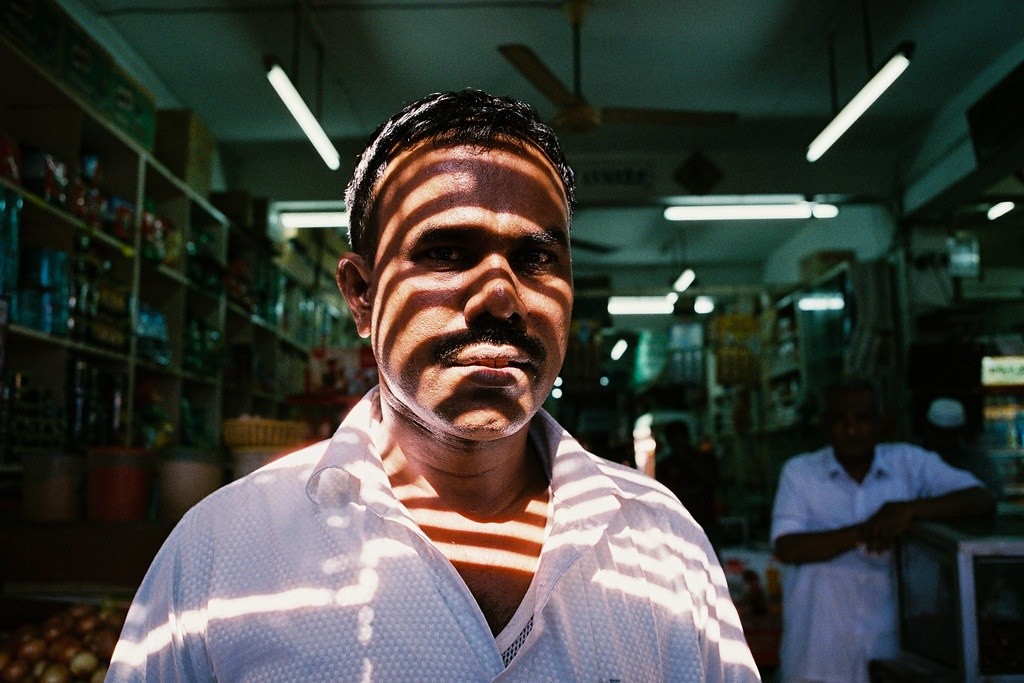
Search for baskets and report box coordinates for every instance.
[225,418,310,446]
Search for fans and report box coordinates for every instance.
[495,0,740,139]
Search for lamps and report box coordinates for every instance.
[265,1,341,173]
[807,0,914,164]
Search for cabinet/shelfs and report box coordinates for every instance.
[709,260,1024,683]
[0,38,375,473]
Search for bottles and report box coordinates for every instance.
[983,395,1024,450]
[70,360,124,448]
[68,237,95,340]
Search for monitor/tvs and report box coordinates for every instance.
[964,59,1024,268]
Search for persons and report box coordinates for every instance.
[105,88,761,681]
[766,371,997,683]
[657,423,721,565]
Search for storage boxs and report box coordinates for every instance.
[157,107,214,201]
[722,541,785,628]
